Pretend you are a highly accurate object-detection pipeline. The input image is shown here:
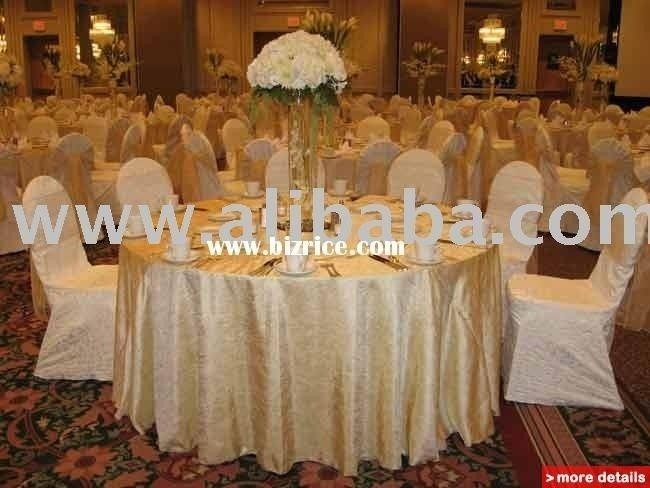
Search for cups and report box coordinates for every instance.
[246,181,260,193]
[334,179,348,193]
[551,119,562,132]
[285,247,310,272]
[407,185,418,197]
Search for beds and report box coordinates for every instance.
[110,195,503,477]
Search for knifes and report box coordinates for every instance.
[372,251,410,273]
[246,257,281,277]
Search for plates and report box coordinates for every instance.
[632,146,650,153]
[317,150,341,158]
[123,188,489,265]
[276,263,317,276]
[0,134,49,150]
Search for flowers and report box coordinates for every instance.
[0,7,623,130]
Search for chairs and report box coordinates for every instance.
[0,85,649,409]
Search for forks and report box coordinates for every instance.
[321,261,341,280]
